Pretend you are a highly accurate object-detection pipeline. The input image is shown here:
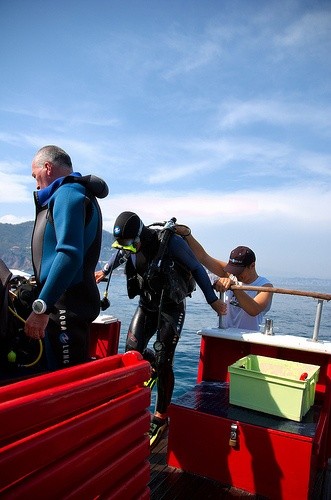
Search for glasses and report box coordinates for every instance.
[111,241,137,255]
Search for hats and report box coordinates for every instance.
[114,211,142,245]
[224,246,256,274]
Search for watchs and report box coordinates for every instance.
[32,299,51,316]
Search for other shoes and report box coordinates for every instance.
[149,416,169,445]
[143,368,160,389]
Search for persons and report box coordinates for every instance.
[24,145,100,371]
[94,212,227,450]
[174,223,273,331]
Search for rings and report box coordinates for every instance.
[224,281,226,284]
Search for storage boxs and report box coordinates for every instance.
[164,378,328,500]
[227,354,321,423]
